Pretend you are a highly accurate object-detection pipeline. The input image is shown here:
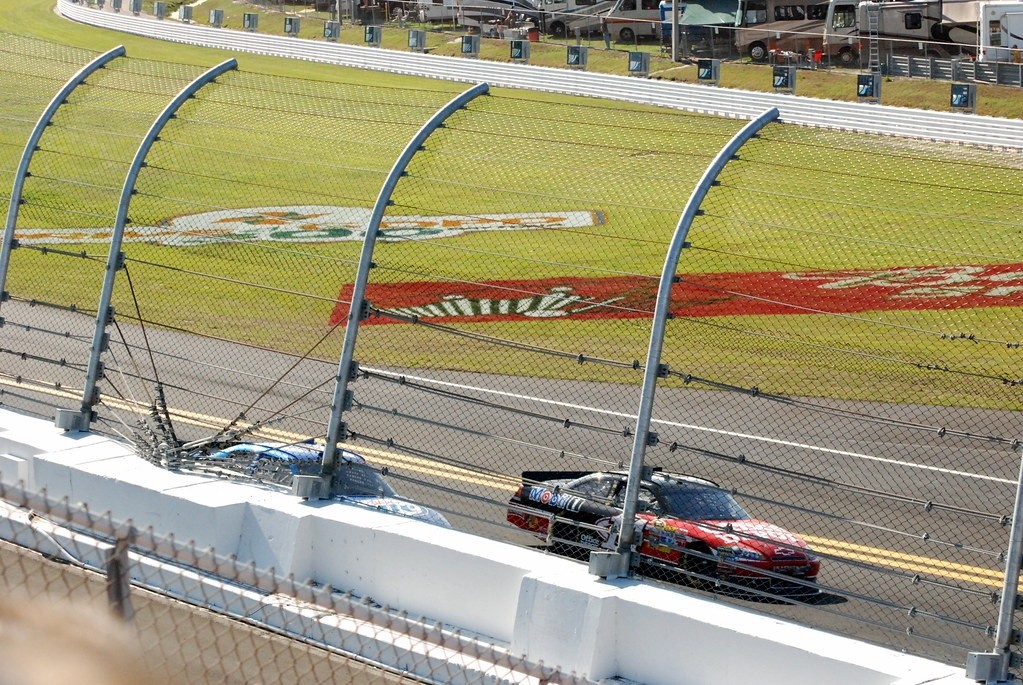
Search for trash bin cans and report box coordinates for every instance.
[528,28,539,42]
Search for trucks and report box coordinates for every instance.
[976,1,1023,64]
[416,0,458,20]
[457,0,538,37]
[539,0,617,36]
[858,0,980,71]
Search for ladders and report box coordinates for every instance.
[866,6,882,74]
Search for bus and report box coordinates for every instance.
[735,1,831,61]
[600,0,659,39]
[824,0,859,64]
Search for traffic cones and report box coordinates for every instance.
[466,26,471,37]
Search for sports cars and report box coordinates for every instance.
[175,440,454,530]
[508,466,822,599]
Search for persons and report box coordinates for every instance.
[1012,45,1021,63]
[601,18,611,50]
[505,5,516,29]
[412,7,418,23]
